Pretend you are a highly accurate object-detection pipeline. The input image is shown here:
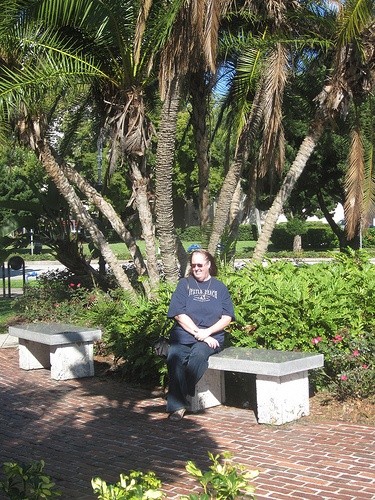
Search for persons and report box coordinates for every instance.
[164,249,235,421]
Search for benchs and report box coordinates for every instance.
[8,322,102,380]
[186,347,323,427]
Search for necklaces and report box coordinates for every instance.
[194,278,213,301]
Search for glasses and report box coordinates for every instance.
[190,261,210,268]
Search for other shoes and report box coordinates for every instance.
[169,408,187,422]
